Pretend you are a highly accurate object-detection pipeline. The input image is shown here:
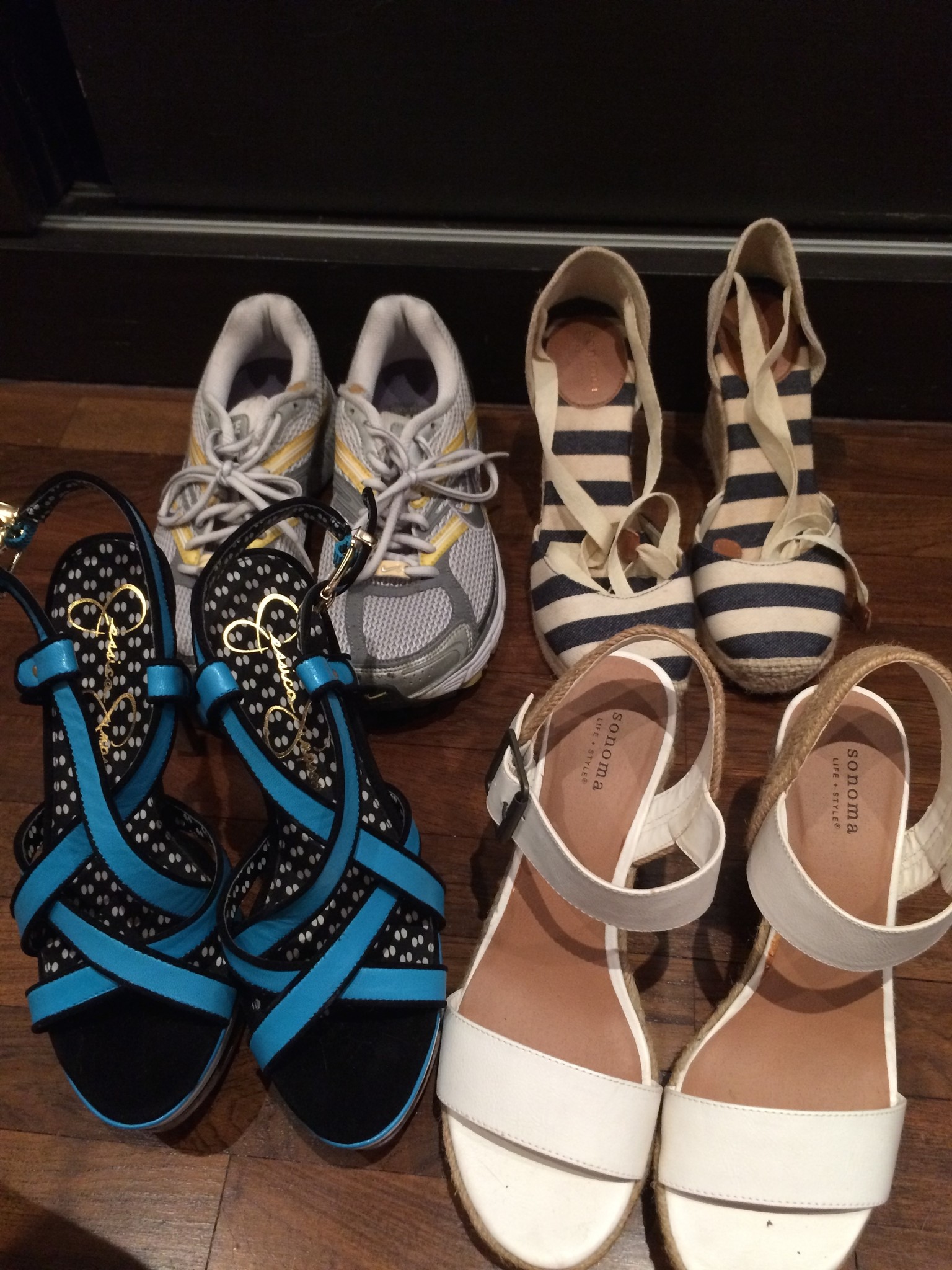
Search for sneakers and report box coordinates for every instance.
[152,291,508,706]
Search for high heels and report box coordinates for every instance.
[523,215,870,696]
[438,625,952,1270]
[0,466,445,1149]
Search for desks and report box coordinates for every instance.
[0,378,950,1270]
